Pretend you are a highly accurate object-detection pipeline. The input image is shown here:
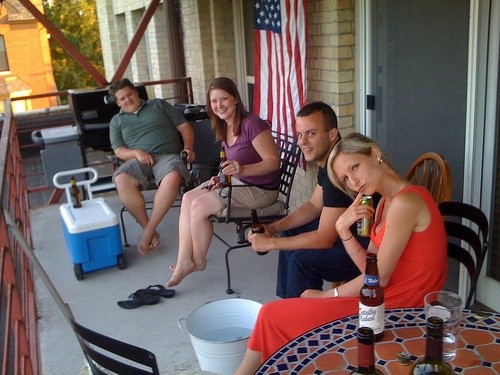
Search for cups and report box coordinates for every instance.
[423,289,463,363]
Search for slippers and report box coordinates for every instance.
[117,285,176,309]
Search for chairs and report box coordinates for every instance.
[121,118,301,295]
[65,303,160,375]
[435,201,489,310]
[404,152,452,207]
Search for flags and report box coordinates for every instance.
[252,0,308,172]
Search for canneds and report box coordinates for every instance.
[357,196,373,237]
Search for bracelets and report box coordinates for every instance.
[333,287,339,297]
[341,233,354,240]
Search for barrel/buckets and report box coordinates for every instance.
[178,298,263,375]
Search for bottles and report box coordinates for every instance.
[358,253,384,342]
[348,326,384,375]
[70,176,82,208]
[409,315,456,375]
[181,152,192,171]
[250,209,269,256]
[218,151,231,188]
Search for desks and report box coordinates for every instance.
[251,307,500,375]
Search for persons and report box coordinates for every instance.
[248,101,382,298]
[233,132,449,375]
[108,78,196,256]
[166,76,280,288]
